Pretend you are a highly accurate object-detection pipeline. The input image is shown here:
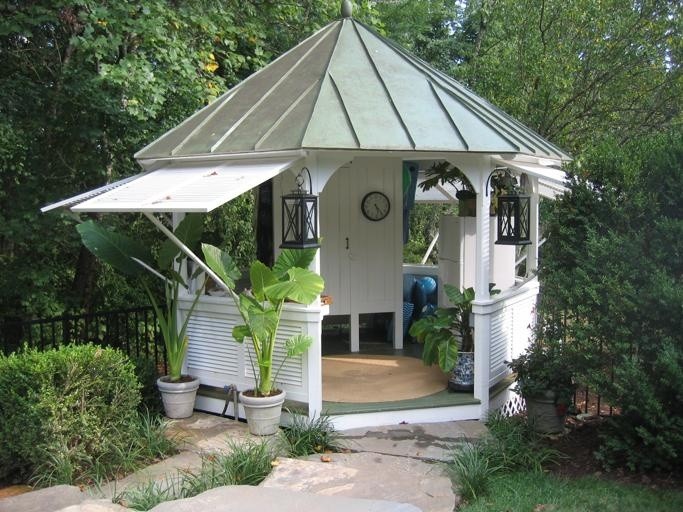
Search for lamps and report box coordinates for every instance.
[276,167,321,250]
[484,165,532,248]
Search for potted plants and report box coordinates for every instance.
[504,344,579,438]
[407,282,501,389]
[77,210,224,419]
[201,244,325,435]
[418,161,512,217]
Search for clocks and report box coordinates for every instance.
[361,191,389,222]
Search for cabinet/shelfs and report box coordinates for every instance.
[274,159,404,354]
